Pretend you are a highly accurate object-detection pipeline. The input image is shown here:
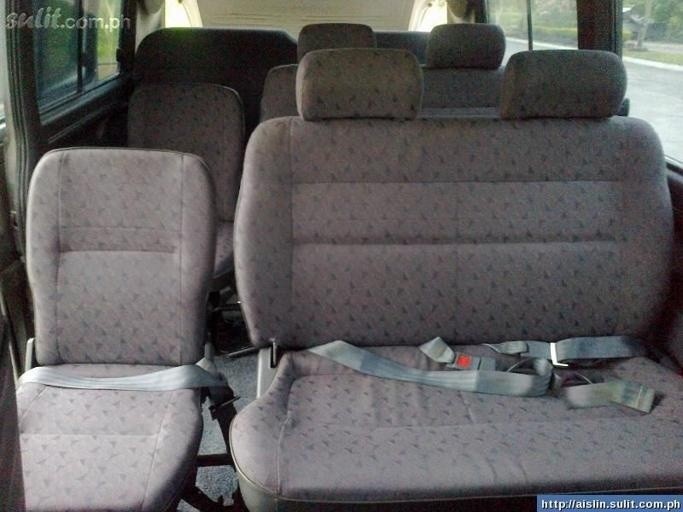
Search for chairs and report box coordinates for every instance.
[129,82,245,280]
[0,147,217,509]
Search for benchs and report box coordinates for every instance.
[229,47,682,508]
[372,29,429,64]
[134,30,297,119]
[259,25,630,123]
